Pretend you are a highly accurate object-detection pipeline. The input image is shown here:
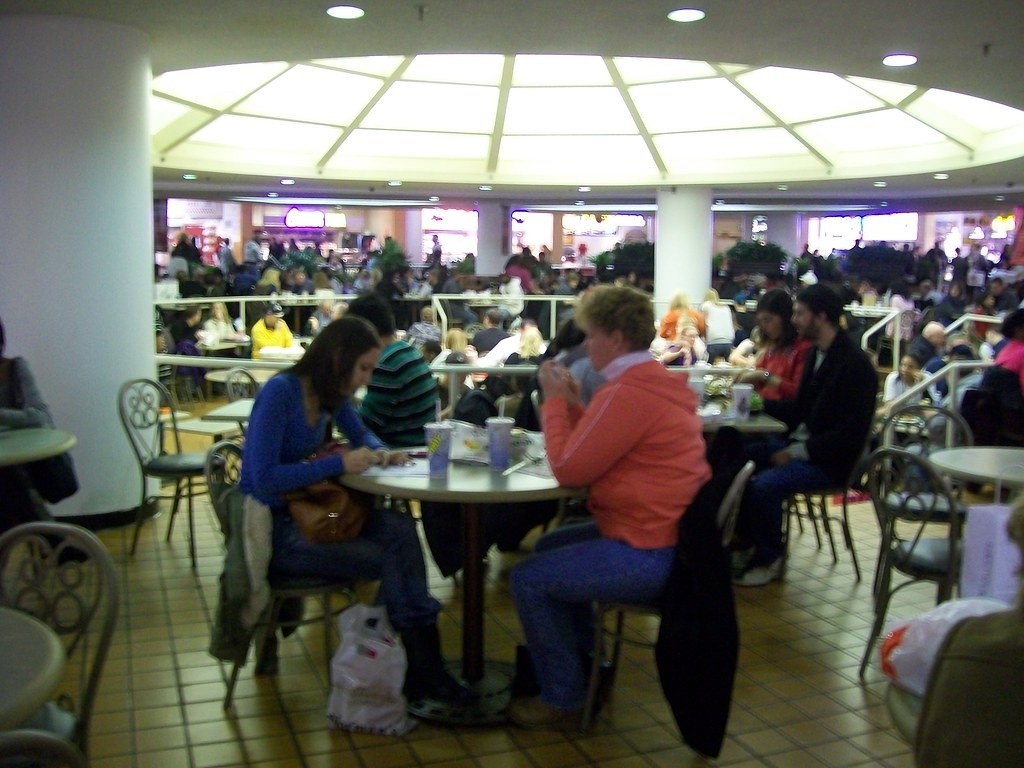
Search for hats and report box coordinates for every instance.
[759,289,794,319]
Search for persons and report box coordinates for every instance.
[169,229,767,396]
[342,289,439,450]
[508,283,712,727]
[796,238,1024,493]
[240,317,481,715]
[1,316,52,573]
[719,283,879,586]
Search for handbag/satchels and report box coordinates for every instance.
[289,440,374,545]
[881,598,1012,698]
[958,490,1024,609]
[25,451,80,505]
[326,604,420,737]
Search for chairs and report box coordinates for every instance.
[204,439,357,710]
[0,521,120,767]
[579,460,755,737]
[226,370,260,402]
[118,377,225,568]
[787,405,972,676]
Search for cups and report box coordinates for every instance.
[732,385,753,422]
[425,421,453,474]
[487,418,514,470]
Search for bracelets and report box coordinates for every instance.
[760,371,772,383]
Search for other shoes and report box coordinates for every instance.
[728,557,784,586]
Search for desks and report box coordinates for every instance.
[202,398,256,434]
[696,413,787,442]
[0,606,66,730]
[164,419,249,443]
[929,447,1024,505]
[204,368,276,383]
[346,452,574,726]
[0,427,78,466]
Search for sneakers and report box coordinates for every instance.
[506,691,581,731]
